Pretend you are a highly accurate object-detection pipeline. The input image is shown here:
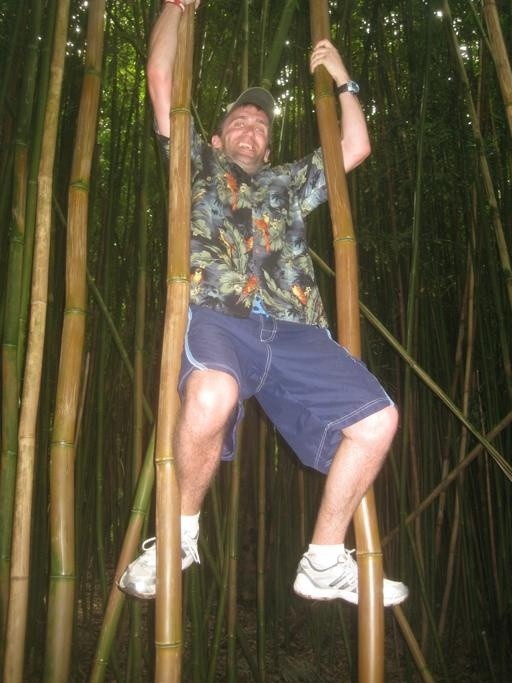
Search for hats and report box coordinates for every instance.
[224,86,276,126]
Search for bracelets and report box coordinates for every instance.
[161,0,185,13]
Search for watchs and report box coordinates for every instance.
[336,81,361,95]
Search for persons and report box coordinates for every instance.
[116,0,410,607]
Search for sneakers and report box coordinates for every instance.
[117,533,200,600]
[293,549,409,608]
